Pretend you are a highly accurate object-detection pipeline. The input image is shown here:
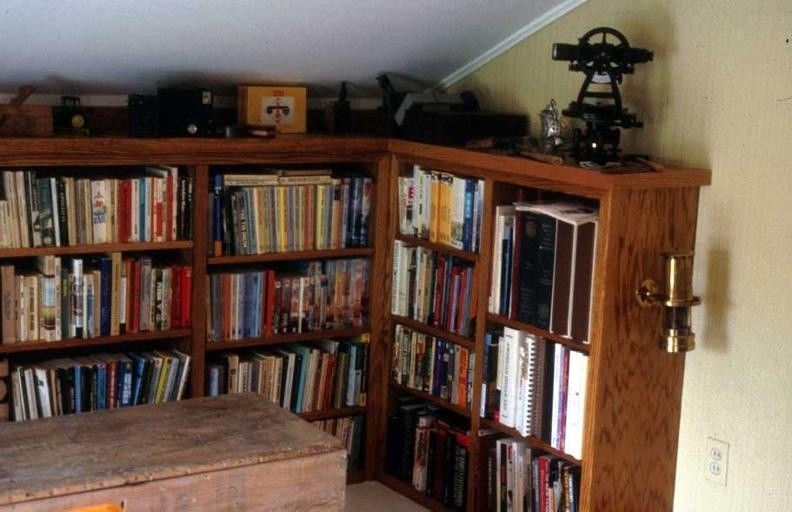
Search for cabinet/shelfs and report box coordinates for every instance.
[386,138,713,511]
[0,137,385,512]
